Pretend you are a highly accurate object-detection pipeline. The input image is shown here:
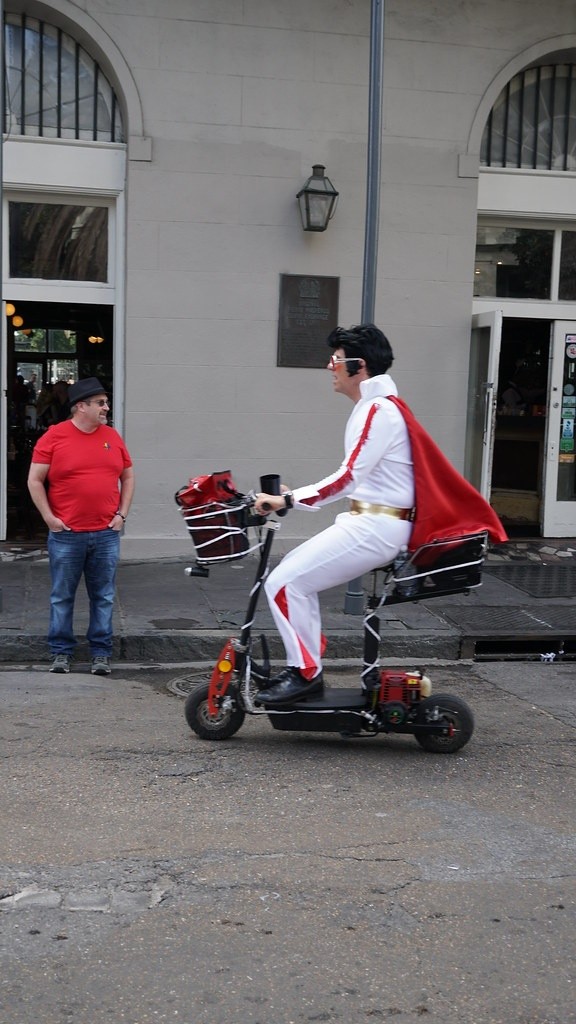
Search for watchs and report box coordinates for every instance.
[281,491,293,510]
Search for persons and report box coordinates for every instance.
[254,324,416,705]
[8,373,113,428]
[27,376,134,674]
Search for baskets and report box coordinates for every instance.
[179,492,249,561]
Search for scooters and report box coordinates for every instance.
[172,472,489,753]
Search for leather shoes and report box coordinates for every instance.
[272,668,295,681]
[255,671,323,705]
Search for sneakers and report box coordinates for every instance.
[91,657,111,675]
[48,654,75,673]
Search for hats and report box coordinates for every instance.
[65,377,109,406]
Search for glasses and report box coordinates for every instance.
[330,356,365,367]
[82,400,109,406]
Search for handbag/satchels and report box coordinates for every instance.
[175,469,244,556]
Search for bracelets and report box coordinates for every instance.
[116,511,126,523]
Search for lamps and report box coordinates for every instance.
[296,163,338,231]
